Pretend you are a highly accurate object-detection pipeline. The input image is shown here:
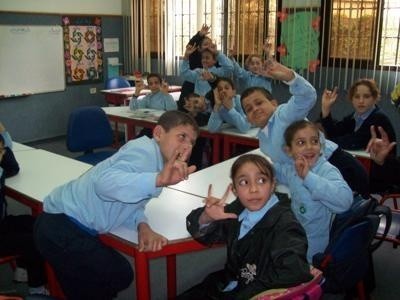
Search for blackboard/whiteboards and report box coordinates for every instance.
[0,25,66,99]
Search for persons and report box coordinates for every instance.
[0,122,12,178]
[33,110,200,300]
[176,49,400,299]
[0,134,32,282]
[130,25,271,167]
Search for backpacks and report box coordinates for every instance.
[325,197,391,255]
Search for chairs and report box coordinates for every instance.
[66,107,118,166]
[318,222,371,300]
[105,77,131,89]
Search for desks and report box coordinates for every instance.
[5,148,226,299]
[166,148,289,299]
[100,85,373,172]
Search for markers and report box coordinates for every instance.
[0,93,32,98]
